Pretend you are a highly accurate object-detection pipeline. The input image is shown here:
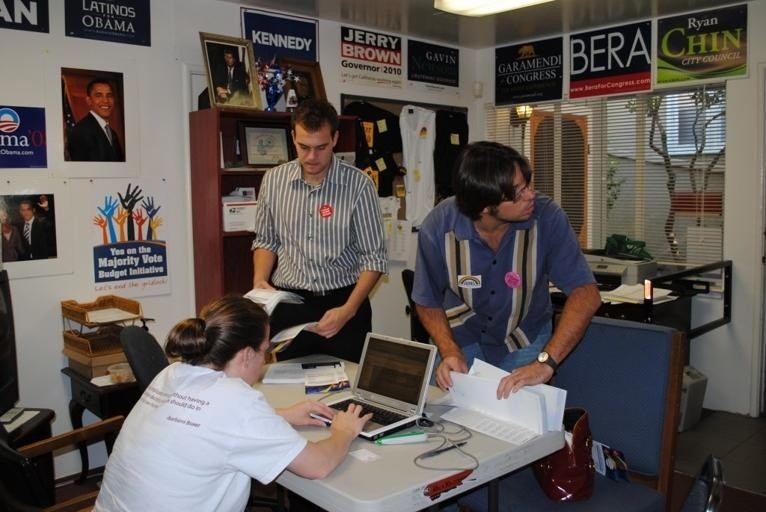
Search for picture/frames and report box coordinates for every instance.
[48,50,138,179]
[283,60,328,110]
[199,31,262,109]
[0,181,72,278]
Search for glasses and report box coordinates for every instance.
[506,181,531,204]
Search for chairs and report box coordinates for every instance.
[456,313,685,512]
[0,417,147,512]
[115,325,170,383]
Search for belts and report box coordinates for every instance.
[289,288,351,300]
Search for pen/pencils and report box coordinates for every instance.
[420,442,467,459]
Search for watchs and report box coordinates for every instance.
[537,351,558,371]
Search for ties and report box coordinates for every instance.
[24,223,29,244]
[105,124,112,142]
[227,66,233,79]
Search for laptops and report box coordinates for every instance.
[309,331,439,443]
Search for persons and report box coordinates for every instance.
[93,296,375,512]
[411,141,601,401]
[0,204,27,262]
[17,195,54,258]
[250,97,389,364]
[69,81,120,159]
[216,49,251,105]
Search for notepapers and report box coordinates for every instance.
[450,134,459,144]
[377,119,387,133]
[375,158,387,171]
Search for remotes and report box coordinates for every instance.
[0,407,24,422]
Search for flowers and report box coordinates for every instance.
[255,57,299,91]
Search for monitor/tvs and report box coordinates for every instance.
[0,269,20,414]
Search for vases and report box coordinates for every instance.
[265,83,284,112]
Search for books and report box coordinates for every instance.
[302,358,351,394]
[264,359,305,384]
[604,282,673,303]
[243,288,304,315]
[271,322,319,341]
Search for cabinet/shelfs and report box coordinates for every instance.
[189,109,360,319]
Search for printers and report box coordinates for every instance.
[583,249,657,292]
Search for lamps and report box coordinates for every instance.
[516,105,533,119]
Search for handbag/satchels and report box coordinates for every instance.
[533,405,595,503]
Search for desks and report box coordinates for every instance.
[252,353,566,511]
